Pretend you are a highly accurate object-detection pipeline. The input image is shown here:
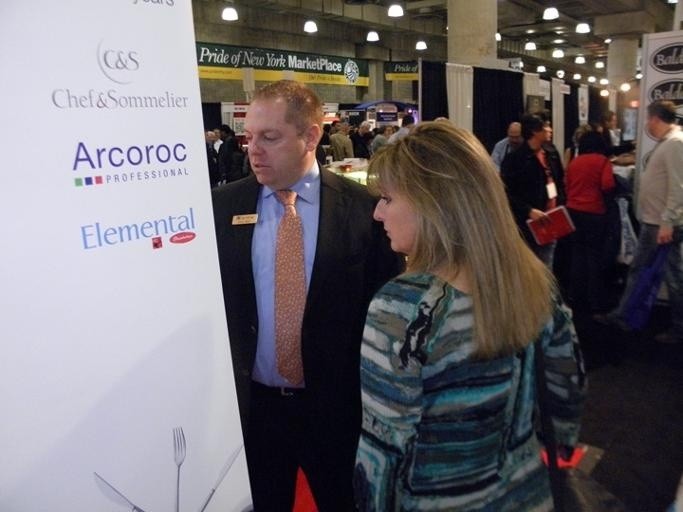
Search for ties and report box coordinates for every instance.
[273,190,307,384]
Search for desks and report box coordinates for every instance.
[323,158,369,185]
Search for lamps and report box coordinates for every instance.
[221,0,427,51]
[496,0,644,97]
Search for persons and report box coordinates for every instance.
[205,123,252,189]
[491,109,637,308]
[211,79,408,512]
[589,98,683,345]
[342,116,589,510]
[316,113,415,166]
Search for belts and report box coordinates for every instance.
[280,386,302,398]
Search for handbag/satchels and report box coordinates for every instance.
[548,460,625,512]
[618,268,665,332]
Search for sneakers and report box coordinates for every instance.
[653,328,683,345]
[591,313,634,333]
